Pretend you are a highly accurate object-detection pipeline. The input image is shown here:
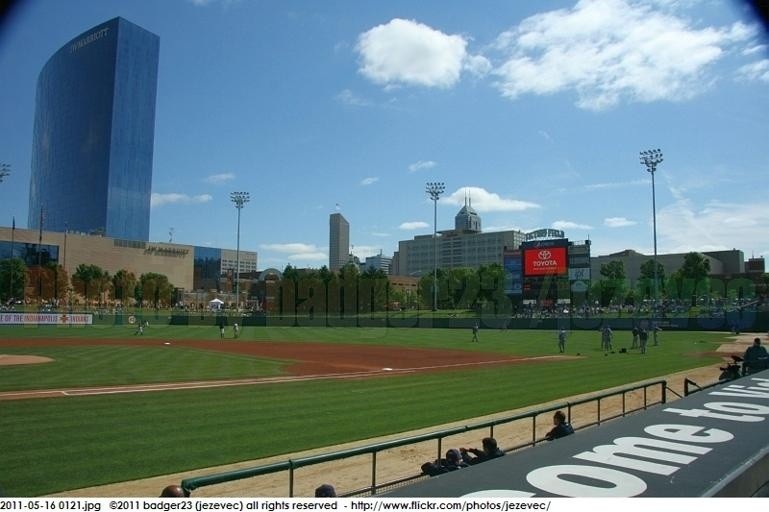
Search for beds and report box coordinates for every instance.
[209,298,225,304]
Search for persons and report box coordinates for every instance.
[739,337,769,376]
[544,410,574,442]
[558,326,566,353]
[420,449,468,477]
[472,320,480,342]
[511,293,769,319]
[600,323,662,355]
[143,319,150,328]
[220,320,225,344]
[314,484,337,497]
[134,322,144,335]
[459,437,506,466]
[232,322,241,337]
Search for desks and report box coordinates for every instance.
[1,161,12,184]
[637,146,663,309]
[227,190,252,312]
[425,181,445,313]
